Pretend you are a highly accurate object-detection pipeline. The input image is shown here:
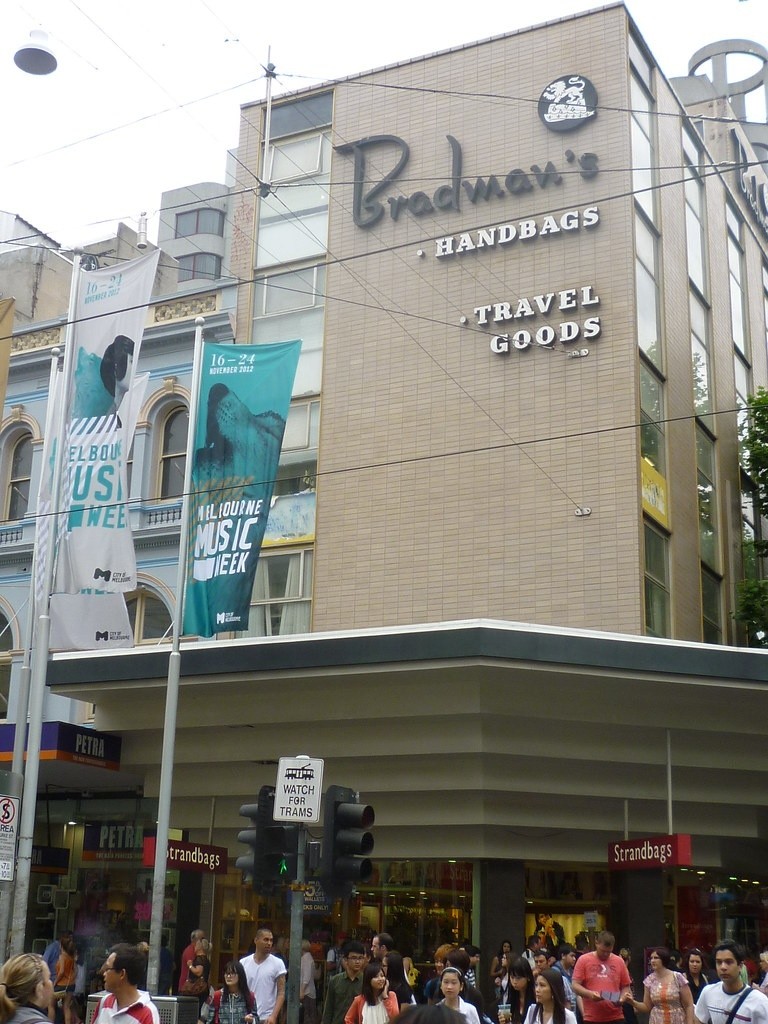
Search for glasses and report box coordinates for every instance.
[105,966,116,973]
[224,972,238,977]
[347,957,364,963]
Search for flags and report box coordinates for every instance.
[32,249,162,649]
[179,339,304,638]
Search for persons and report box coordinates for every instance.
[496,957,536,1024]
[178,930,213,1019]
[434,967,481,1024]
[300,939,316,1024]
[239,928,288,1024]
[0,953,55,1024]
[524,968,577,1024]
[572,931,632,1024]
[345,964,399,1024]
[321,942,365,1024]
[43,930,85,1024]
[694,945,768,1024]
[90,943,160,1024]
[95,336,135,432]
[533,912,564,948]
[198,961,258,1024]
[369,933,768,1024]
[385,1004,469,1024]
[625,947,694,1024]
[146,934,173,997]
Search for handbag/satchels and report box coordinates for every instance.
[405,957,419,988]
[180,955,208,996]
[54,990,66,998]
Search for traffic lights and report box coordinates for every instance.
[235,785,281,896]
[321,784,376,898]
[262,825,300,884]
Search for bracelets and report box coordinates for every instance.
[189,965,192,969]
